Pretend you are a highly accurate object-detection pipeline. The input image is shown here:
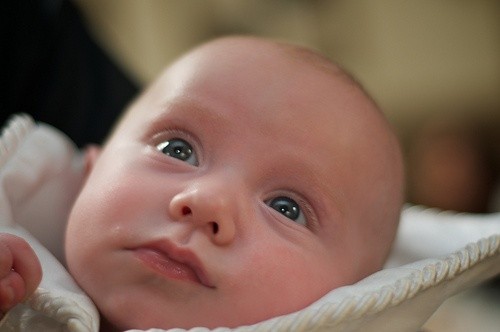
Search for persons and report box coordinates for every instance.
[1,34,411,332]
